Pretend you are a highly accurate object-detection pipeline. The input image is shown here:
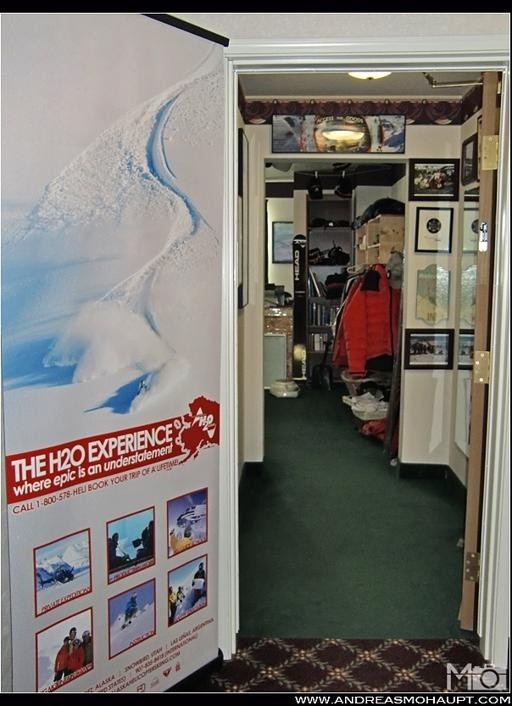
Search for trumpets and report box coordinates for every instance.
[292,235,310,382]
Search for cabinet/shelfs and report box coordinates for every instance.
[305,189,405,384]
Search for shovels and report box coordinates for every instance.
[312,341,332,387]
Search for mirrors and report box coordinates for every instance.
[451,187,479,461]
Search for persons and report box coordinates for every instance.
[121,591,138,629]
[168,584,185,621]
[190,562,204,605]
[412,342,436,355]
[175,529,194,553]
[414,169,447,191]
[52,627,93,682]
[109,533,128,569]
[136,520,155,559]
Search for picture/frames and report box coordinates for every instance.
[404,158,461,253]
[461,131,478,185]
[458,329,475,370]
[271,220,295,265]
[404,328,455,370]
[462,208,479,254]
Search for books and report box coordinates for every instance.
[307,266,340,351]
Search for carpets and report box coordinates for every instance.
[181,638,509,694]
